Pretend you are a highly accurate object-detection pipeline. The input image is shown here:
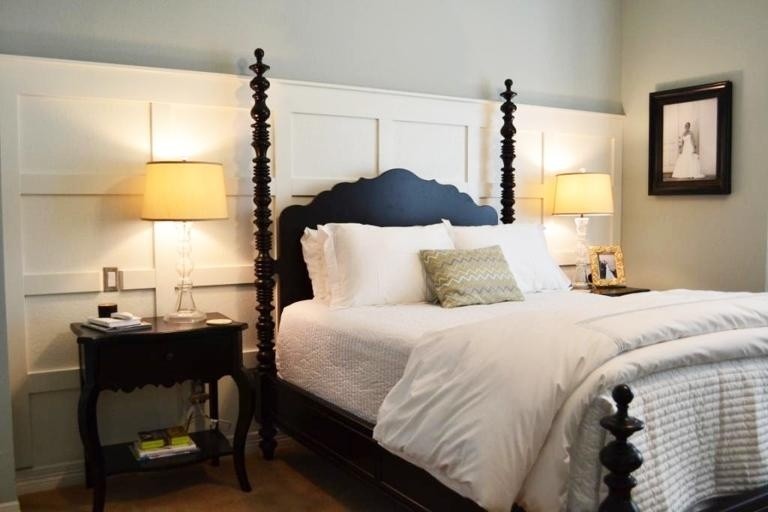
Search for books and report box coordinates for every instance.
[129,425,200,461]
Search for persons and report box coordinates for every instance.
[671,121,706,178]
[600,260,616,280]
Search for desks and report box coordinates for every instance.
[569,285,650,296]
[71,312,255,512]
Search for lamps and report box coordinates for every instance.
[552,171,614,292]
[141,160,228,324]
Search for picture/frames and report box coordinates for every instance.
[589,245,626,288]
[648,81,732,196]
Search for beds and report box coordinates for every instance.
[250,48,768,511]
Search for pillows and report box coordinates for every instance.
[300,219,574,309]
